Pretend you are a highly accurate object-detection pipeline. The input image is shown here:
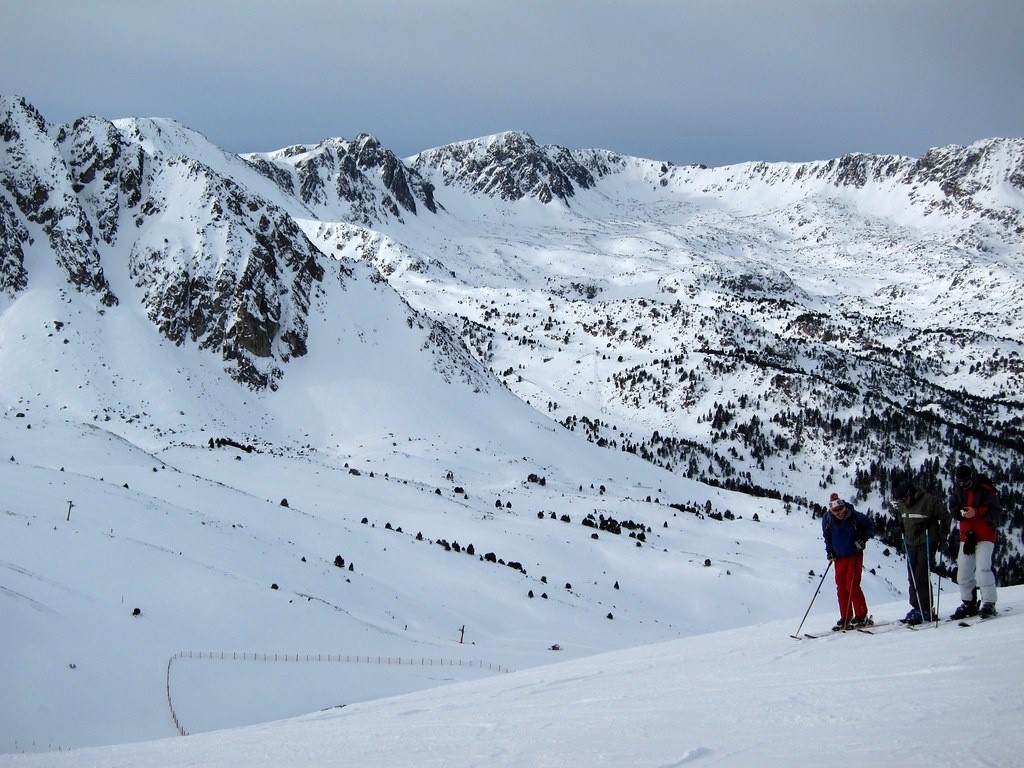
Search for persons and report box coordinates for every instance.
[948,465,1003,619]
[822,493,876,631]
[893,481,953,625]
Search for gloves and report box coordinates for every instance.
[825,548,837,561]
[938,540,948,553]
[896,547,903,555]
[855,534,869,550]
[963,528,980,556]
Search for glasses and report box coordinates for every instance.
[894,496,907,503]
[832,505,846,516]
[956,479,971,489]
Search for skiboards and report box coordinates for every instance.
[856,620,927,635]
[790,621,895,640]
[906,605,1014,631]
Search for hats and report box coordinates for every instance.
[892,480,909,498]
[956,466,973,481]
[829,493,844,511]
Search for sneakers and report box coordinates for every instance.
[905,607,936,622]
[844,616,869,632]
[832,617,854,632]
[978,601,996,619]
[956,600,977,618]
